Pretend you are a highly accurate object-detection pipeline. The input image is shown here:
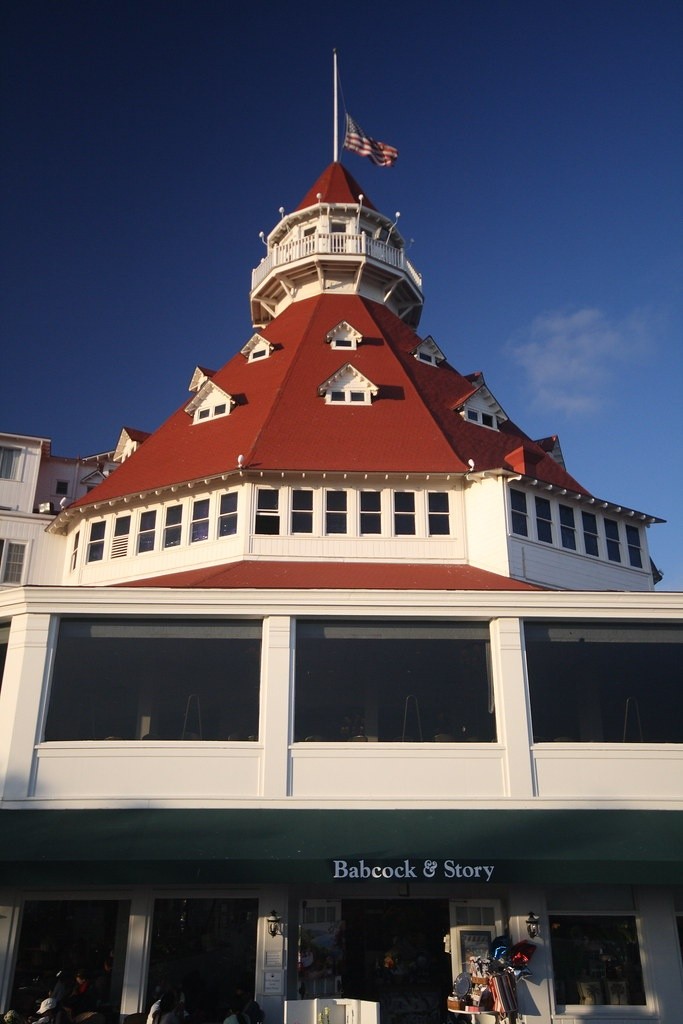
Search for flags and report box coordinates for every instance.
[342,112,399,169]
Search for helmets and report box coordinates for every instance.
[3,1010,19,1024]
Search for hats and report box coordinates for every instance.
[36,997,56,1015]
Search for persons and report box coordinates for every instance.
[0,948,113,1024]
[144,966,264,1024]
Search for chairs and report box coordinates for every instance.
[296,733,458,742]
[105,729,257,741]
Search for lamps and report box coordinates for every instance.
[266,911,281,937]
[238,454,245,468]
[469,458,477,472]
[525,911,539,939]
[61,497,67,511]
